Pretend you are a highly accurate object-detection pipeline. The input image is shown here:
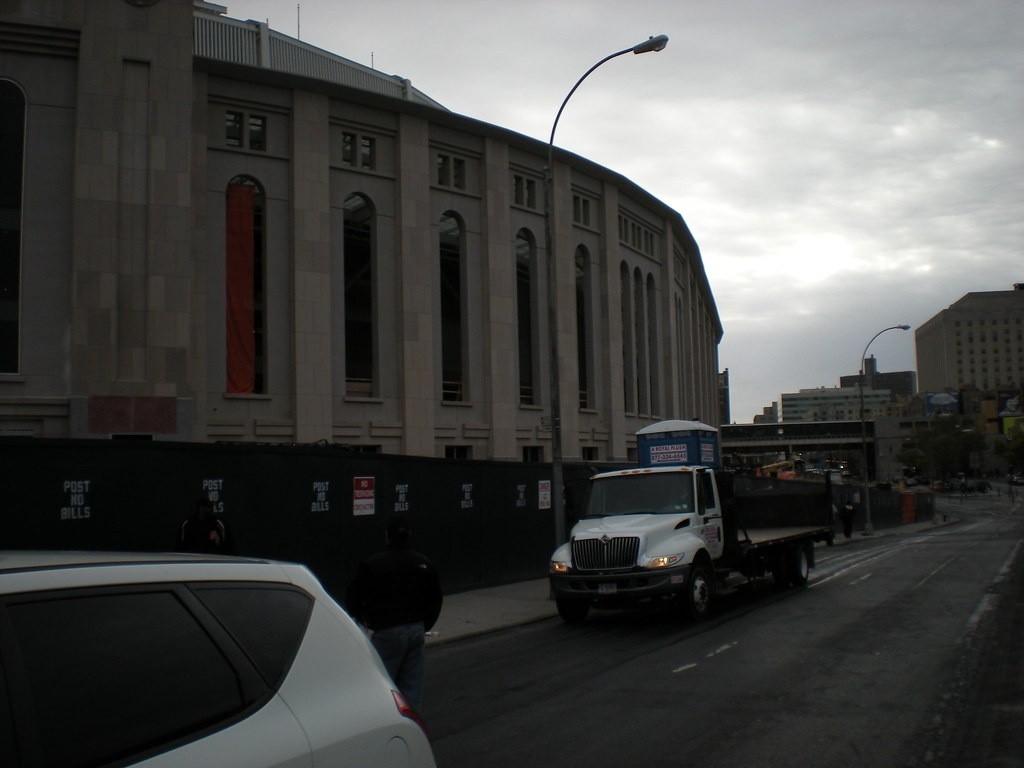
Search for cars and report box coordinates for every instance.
[0,550,438,768]
[930,479,992,494]
[1006,471,1024,485]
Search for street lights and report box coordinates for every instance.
[545,35,670,547]
[962,429,973,498]
[859,325,910,535]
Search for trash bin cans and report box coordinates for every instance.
[899,493,917,524]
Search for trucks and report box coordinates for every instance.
[548,464,834,629]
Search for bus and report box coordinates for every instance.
[806,468,841,483]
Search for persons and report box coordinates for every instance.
[181,499,227,553]
[839,500,856,537]
[349,519,442,712]
